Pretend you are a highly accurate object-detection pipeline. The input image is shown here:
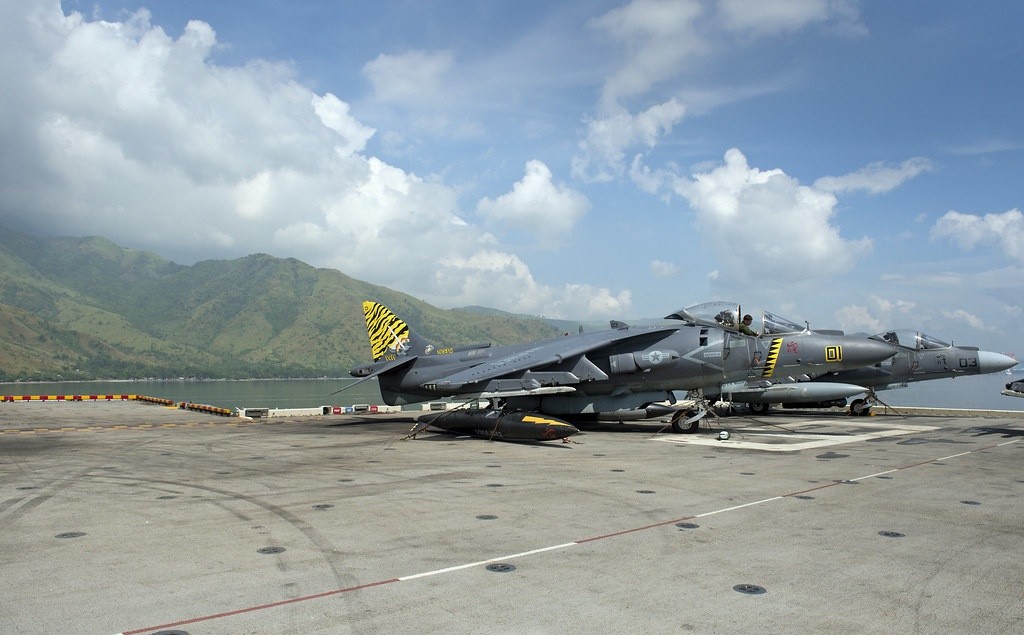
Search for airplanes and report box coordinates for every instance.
[730,310,1021,418]
[326,299,899,445]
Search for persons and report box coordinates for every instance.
[734,315,758,336]
